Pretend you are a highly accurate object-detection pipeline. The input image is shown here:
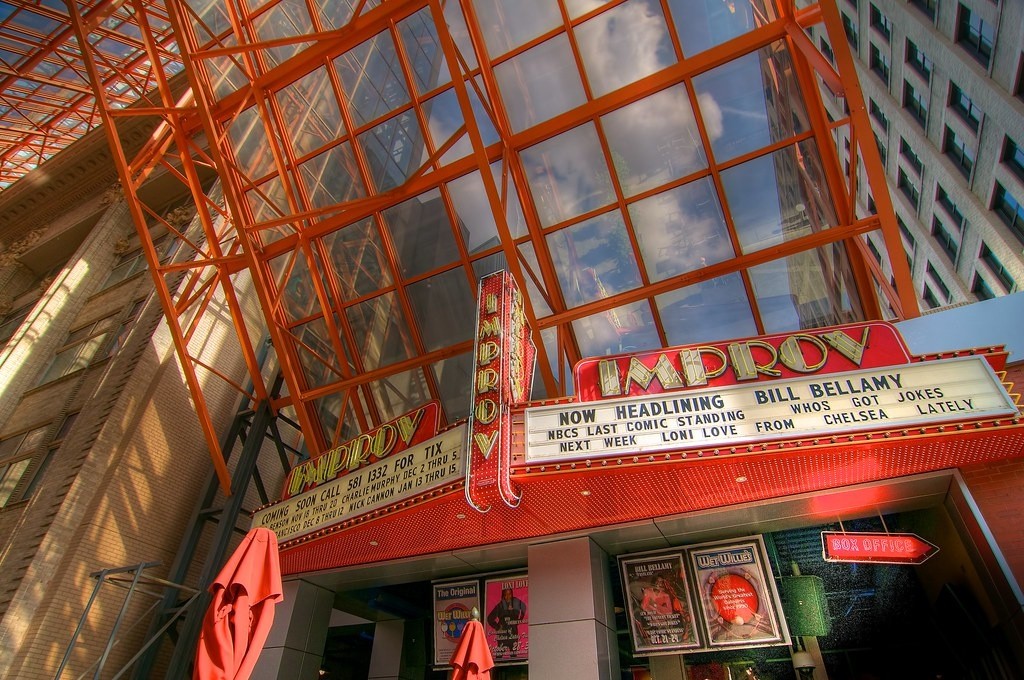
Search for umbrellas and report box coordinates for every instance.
[193,527,286,680]
[449,608,494,680]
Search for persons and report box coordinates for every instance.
[488,586,526,658]
[641,573,673,637]
